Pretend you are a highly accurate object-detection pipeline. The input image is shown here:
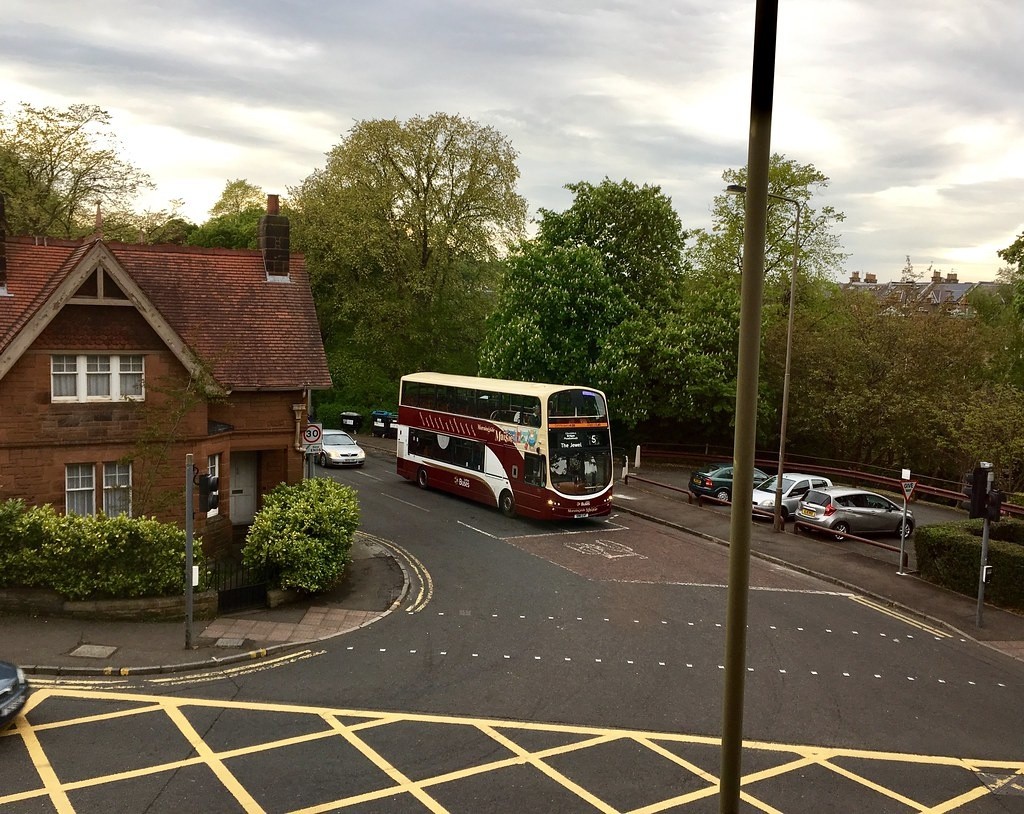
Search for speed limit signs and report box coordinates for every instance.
[302,424,322,444]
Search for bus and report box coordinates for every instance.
[395,372,627,522]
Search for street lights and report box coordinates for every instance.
[726,184,801,533]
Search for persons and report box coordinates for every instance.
[522,414,528,425]
[529,405,541,428]
[548,401,555,423]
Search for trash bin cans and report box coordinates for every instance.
[340,411,363,435]
[371,410,398,439]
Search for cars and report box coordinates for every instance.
[751,474,834,521]
[794,486,915,542]
[316,429,366,468]
[689,462,773,505]
[0,660,30,729]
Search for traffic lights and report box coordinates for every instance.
[986,491,1002,522]
[200,474,219,513]
